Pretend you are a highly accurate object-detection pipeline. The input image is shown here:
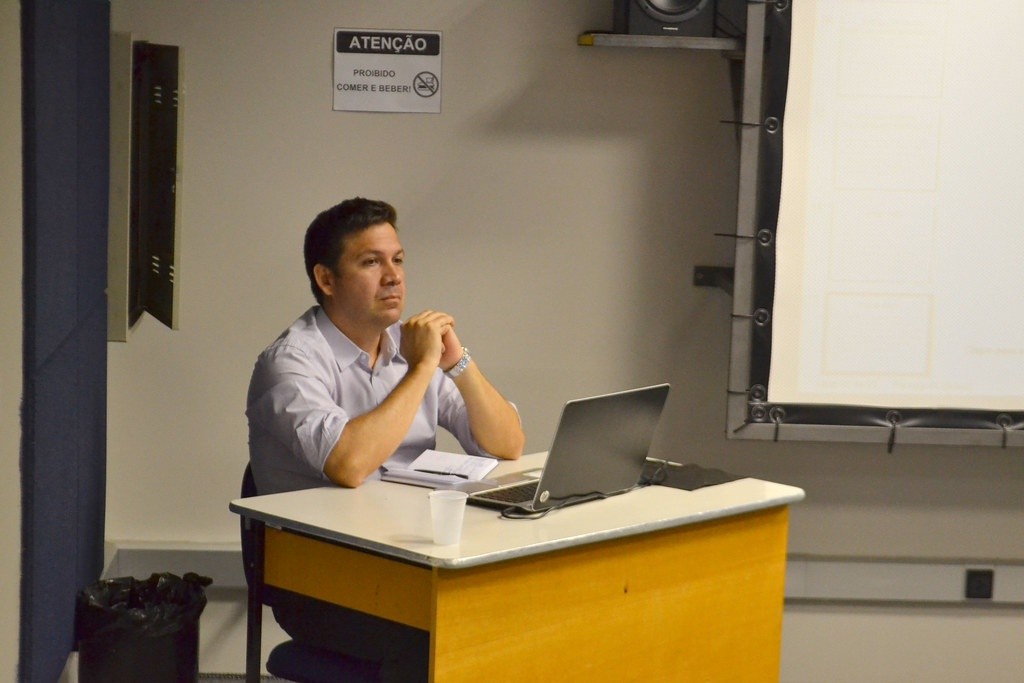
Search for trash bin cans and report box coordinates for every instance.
[81,577,208,683]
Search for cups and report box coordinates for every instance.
[429,491,469,546]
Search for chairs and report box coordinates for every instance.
[240,464,272,683]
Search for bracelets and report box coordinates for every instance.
[443,346,471,376]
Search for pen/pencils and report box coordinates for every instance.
[413,468,469,479]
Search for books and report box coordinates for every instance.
[380,449,499,490]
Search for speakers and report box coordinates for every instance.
[613,0,717,39]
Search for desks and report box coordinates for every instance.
[230,451,805,683]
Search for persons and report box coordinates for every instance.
[244,197,524,683]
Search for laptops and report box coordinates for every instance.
[433,384,671,514]
